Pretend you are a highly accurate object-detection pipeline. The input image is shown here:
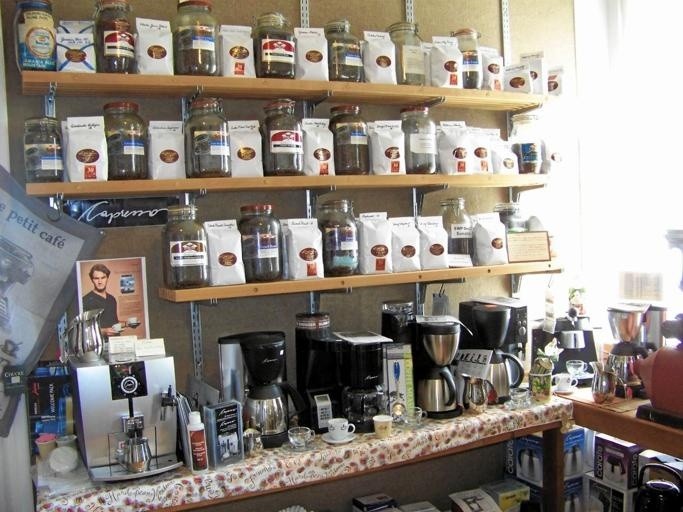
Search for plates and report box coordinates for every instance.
[321,432,357,445]
[505,398,537,412]
[551,382,578,395]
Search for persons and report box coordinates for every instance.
[82,263,140,337]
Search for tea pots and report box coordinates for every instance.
[60,306,107,364]
[590,361,628,404]
[459,372,497,414]
[631,462,683,512]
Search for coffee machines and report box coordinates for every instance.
[605,302,665,398]
[294,312,387,437]
[69,334,183,488]
[458,305,527,406]
[532,307,600,387]
[412,314,461,418]
[215,329,306,454]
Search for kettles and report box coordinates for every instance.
[605,453,628,483]
[587,491,610,512]
[564,446,584,476]
[564,492,580,512]
[519,447,541,483]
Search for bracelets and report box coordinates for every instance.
[125,321,127,327]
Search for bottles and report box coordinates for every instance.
[186,411,210,476]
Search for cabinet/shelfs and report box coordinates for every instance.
[33,399,573,511]
[535,358,681,510]
[19,70,562,383]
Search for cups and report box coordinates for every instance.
[327,418,356,440]
[56,435,79,455]
[397,406,427,426]
[565,360,588,376]
[555,372,577,388]
[373,414,394,438]
[287,426,315,449]
[508,388,535,406]
[34,432,57,463]
